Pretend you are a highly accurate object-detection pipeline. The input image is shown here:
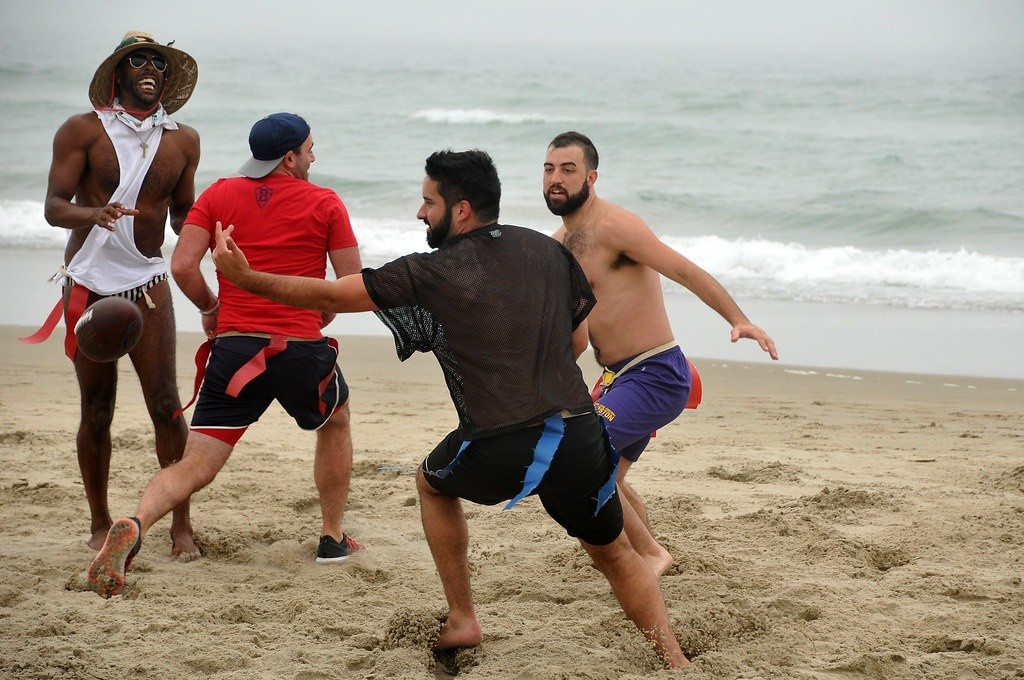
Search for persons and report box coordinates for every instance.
[212,149,691,669]
[44,32,202,562]
[87,113,361,598]
[543,132,779,575]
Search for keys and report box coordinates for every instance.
[140,142,148,158]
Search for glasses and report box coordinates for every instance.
[128,52,168,72]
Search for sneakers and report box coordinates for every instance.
[315,532,365,565]
[86,517,141,599]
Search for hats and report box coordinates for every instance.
[89,31,198,116]
[239,112,310,178]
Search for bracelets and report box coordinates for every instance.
[203,300,220,316]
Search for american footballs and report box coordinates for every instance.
[74,295,146,364]
